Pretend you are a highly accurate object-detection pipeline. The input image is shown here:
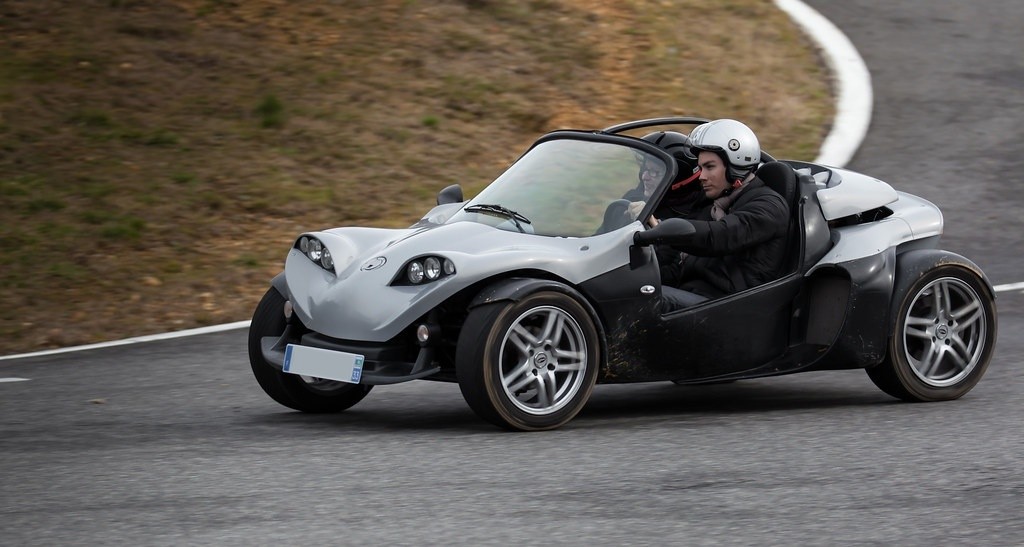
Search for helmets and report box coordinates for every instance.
[632,131,696,190]
[683,117,762,186]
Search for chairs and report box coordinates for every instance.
[756,159,799,220]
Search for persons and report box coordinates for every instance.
[624,119,789,309]
[596,131,704,233]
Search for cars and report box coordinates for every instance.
[248,119,998,433]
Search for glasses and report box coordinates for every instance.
[640,165,668,178]
